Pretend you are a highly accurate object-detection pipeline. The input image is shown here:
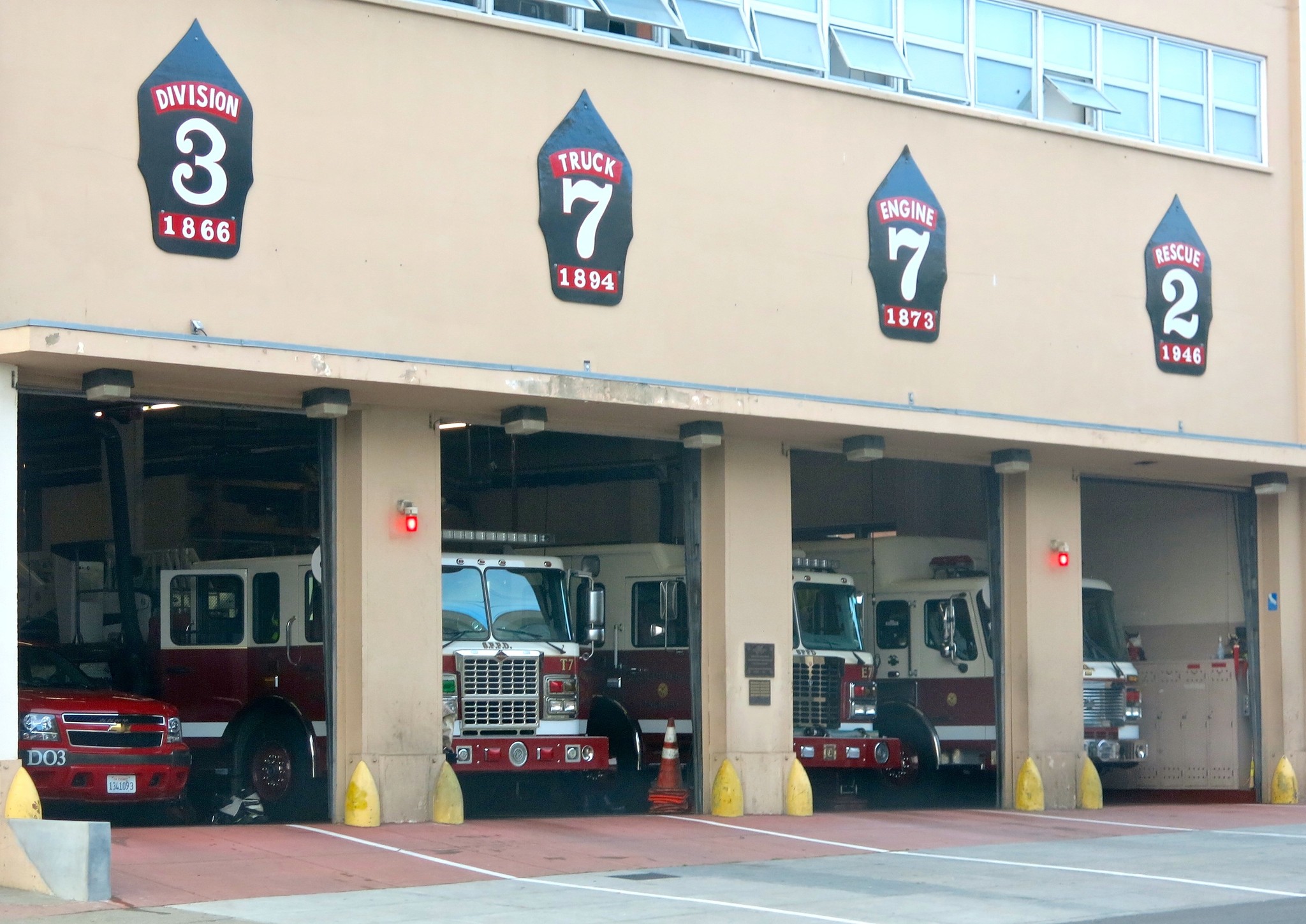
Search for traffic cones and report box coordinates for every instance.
[647,717,689,811]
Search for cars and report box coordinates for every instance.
[18,638,191,812]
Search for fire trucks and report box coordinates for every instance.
[162,531,617,817]
[502,541,902,806]
[796,535,1145,802]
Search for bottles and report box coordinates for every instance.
[1216,637,1224,659]
